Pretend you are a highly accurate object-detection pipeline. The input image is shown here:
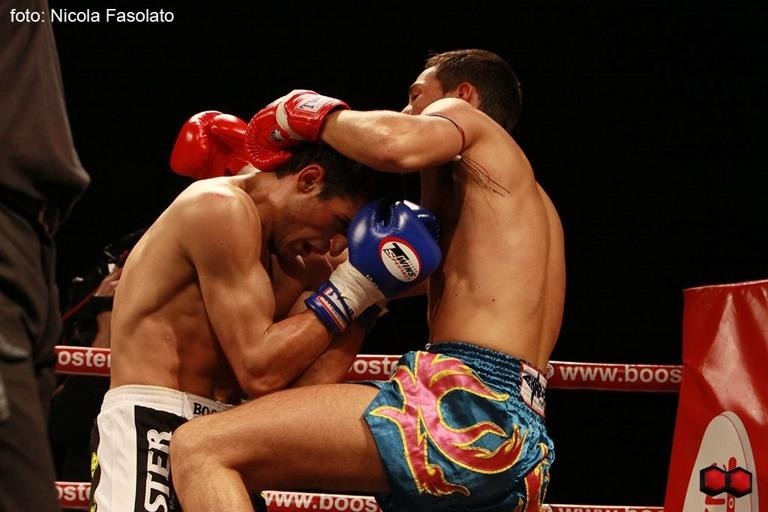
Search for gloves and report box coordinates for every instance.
[170,103,262,182]
[242,85,348,174]
[302,195,445,336]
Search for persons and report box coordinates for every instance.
[170,50,566,511]
[90,140,442,510]
[51,270,121,509]
[0,1,92,508]
